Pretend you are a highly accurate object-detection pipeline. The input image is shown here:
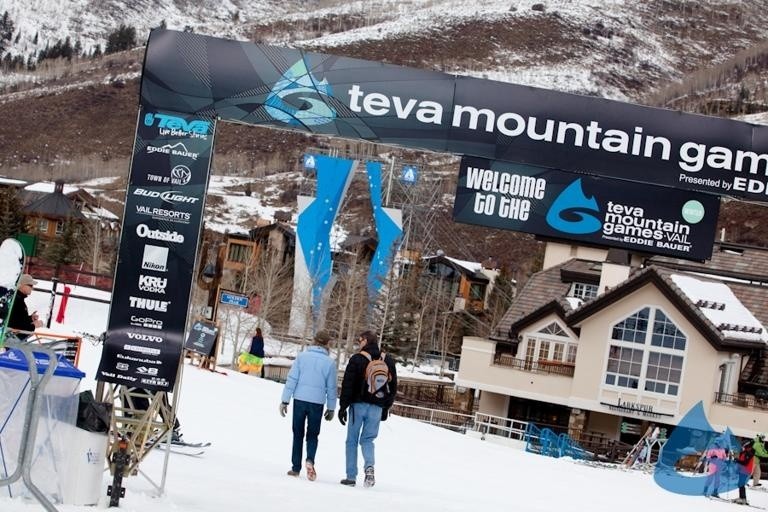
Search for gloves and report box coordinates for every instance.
[338,408,347,425]
[280,403,288,416]
[324,410,334,421]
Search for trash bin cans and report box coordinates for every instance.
[0,347,113,507]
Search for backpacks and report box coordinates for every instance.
[363,352,393,405]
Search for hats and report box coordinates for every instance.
[20,274,38,285]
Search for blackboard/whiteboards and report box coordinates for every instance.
[184,321,219,355]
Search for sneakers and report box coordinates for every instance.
[341,480,355,486]
[288,470,298,475]
[306,461,316,481]
[364,466,375,486]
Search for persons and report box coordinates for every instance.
[0,274,67,357]
[338,330,397,488]
[238,327,264,377]
[120,384,181,445]
[279,329,338,482]
[702,433,768,505]
